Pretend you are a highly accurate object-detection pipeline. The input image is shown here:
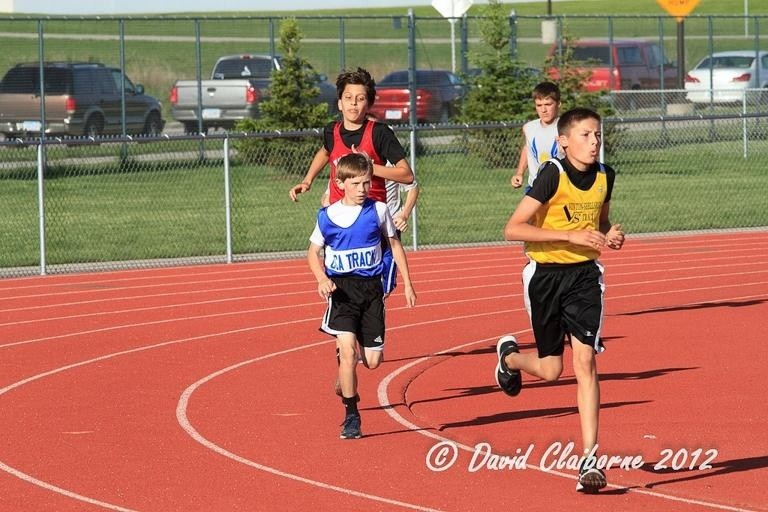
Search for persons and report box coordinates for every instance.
[319,112,420,300]
[304,152,418,439]
[495,107,625,494]
[287,67,414,398]
[509,82,562,196]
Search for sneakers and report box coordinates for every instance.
[495,335,522,397]
[339,412,362,438]
[576,458,606,493]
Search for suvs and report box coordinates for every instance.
[545,37,679,111]
[0,60,165,143]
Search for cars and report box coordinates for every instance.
[685,50,768,106]
[462,66,543,91]
[366,68,470,124]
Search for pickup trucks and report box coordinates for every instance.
[170,54,338,134]
